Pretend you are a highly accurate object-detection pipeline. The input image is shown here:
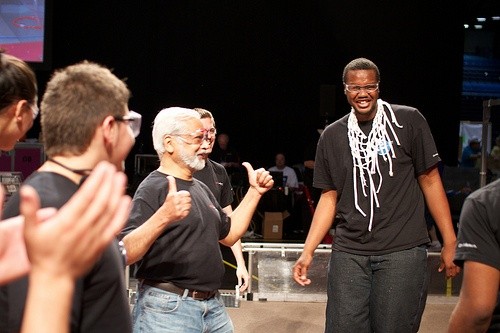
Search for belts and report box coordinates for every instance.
[138,277,218,301]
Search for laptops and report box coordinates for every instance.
[269,171,288,190]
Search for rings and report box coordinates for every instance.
[451,267,455,269]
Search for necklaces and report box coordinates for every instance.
[347,98,404,232]
[46,156,92,178]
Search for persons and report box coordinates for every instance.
[291,56,462,333]
[461,136,500,169]
[445,178,500,333]
[0,51,315,333]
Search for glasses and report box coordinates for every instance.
[202,129,216,136]
[170,133,209,145]
[114,110,141,137]
[344,83,379,93]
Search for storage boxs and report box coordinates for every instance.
[257,210,290,239]
